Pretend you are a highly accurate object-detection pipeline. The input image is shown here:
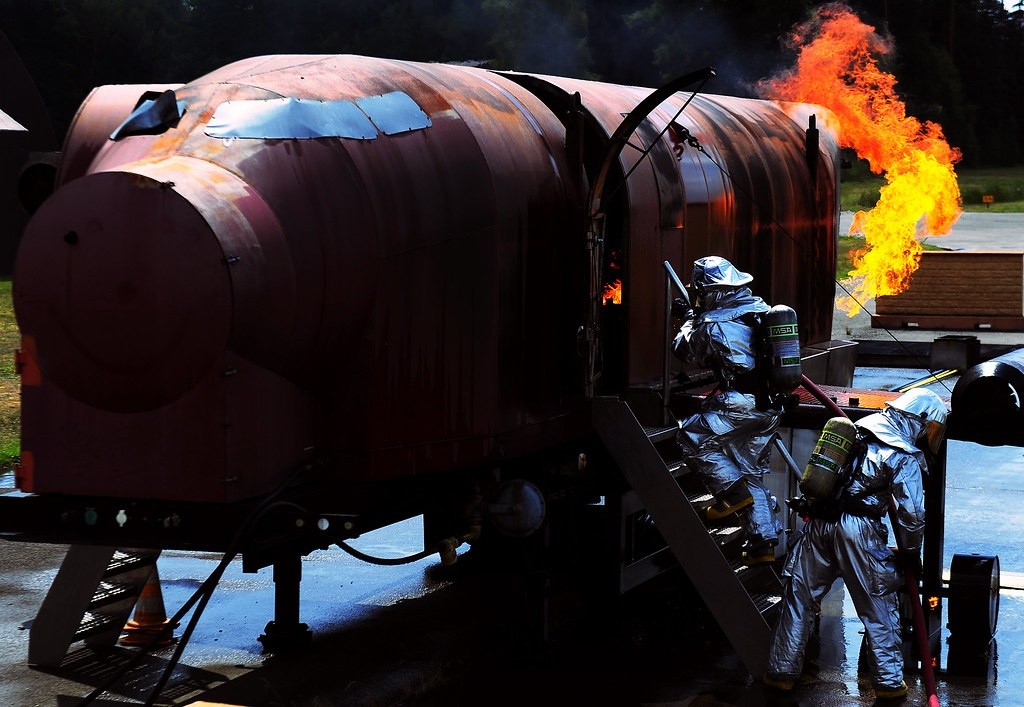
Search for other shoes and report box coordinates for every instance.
[763,672,793,689]
[736,539,775,566]
[874,680,908,698]
[705,481,753,520]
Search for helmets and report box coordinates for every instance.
[884,388,947,425]
[684,256,753,286]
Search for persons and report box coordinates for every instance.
[754,387,947,707]
[672,255,782,564]
